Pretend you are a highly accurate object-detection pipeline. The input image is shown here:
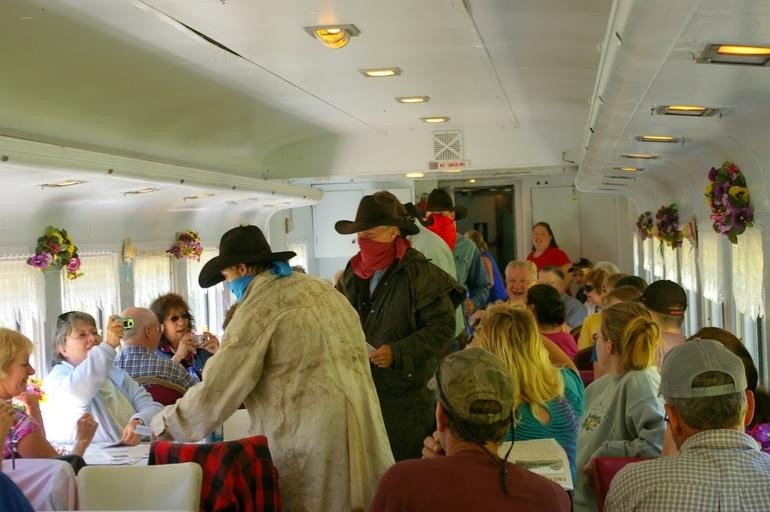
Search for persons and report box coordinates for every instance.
[374,259,770,512]
[152,225,397,512]
[334,188,506,461]
[526,222,571,271]
[1,292,220,512]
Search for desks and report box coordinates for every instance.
[56,440,202,466]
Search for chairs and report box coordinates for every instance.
[131,374,186,406]
[149,436,282,511]
[0,459,75,512]
[568,323,658,512]
[74,461,204,510]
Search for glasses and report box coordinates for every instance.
[586,285,595,292]
[171,313,190,321]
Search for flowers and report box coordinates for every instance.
[635,211,656,241]
[654,203,682,251]
[26,226,84,278]
[703,161,755,245]
[169,228,205,263]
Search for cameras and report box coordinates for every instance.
[115,316,136,338]
[192,334,208,346]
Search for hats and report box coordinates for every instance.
[427,347,514,426]
[335,188,468,235]
[568,258,593,273]
[199,226,296,288]
[631,280,687,315]
[657,337,748,398]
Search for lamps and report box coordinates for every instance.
[305,24,361,48]
[682,215,698,248]
[123,238,137,265]
